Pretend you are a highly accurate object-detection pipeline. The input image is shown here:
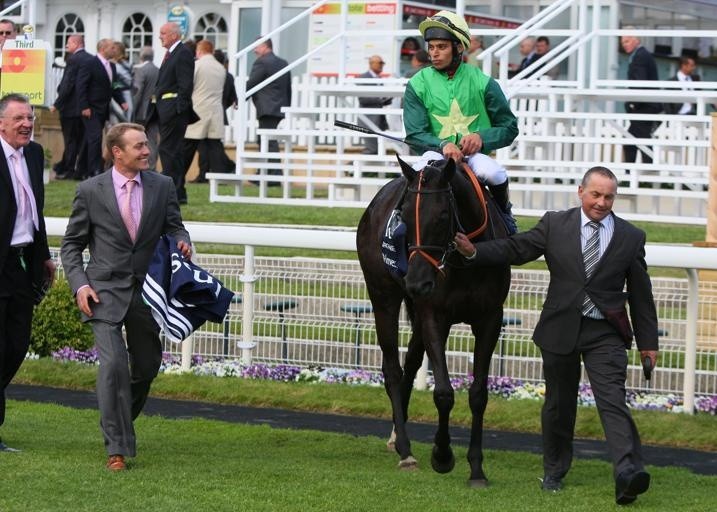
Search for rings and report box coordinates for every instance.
[187,248,192,254]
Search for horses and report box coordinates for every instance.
[355,152,512,489]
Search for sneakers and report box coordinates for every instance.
[108,456,125,470]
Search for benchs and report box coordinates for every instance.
[202,76,717,231]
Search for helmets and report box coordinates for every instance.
[419,10,471,48]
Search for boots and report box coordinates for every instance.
[489,177,516,233]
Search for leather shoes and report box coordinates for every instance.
[55,170,103,181]
[615,469,650,504]
[542,475,562,489]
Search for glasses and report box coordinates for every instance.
[0,31,11,35]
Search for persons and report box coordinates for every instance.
[400,49,428,109]
[451,165,660,505]
[621,25,661,163]
[518,36,561,82]
[347,57,392,176]
[58,122,193,473]
[666,56,702,116]
[0,92,55,454]
[53,22,292,208]
[465,33,499,80]
[0,20,17,46]
[398,7,523,232]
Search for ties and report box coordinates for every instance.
[122,181,136,239]
[583,222,601,316]
[13,152,40,232]
[162,51,170,64]
